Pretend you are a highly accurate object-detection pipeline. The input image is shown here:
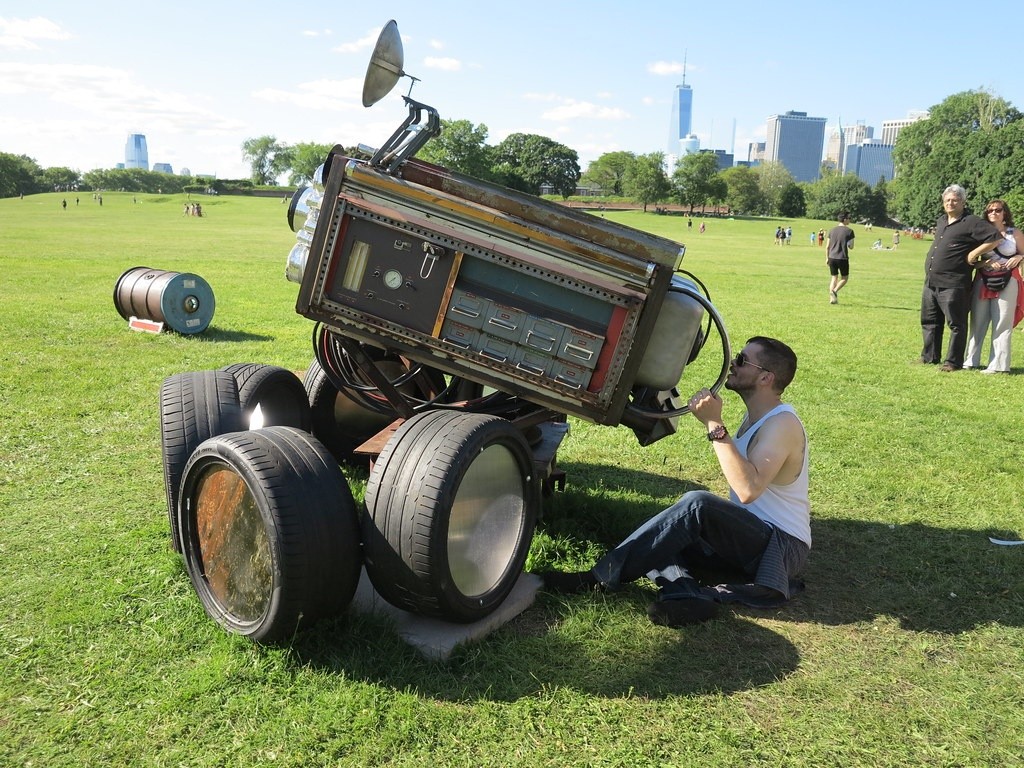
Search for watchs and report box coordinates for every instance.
[708,426,729,441]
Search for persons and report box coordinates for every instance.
[540,337,814,626]
[870,239,892,250]
[905,226,923,240]
[910,183,1024,374]
[826,212,855,304]
[699,221,705,235]
[892,231,900,251]
[687,219,692,232]
[62,193,103,211]
[810,232,816,245]
[818,228,824,247]
[655,206,734,218]
[775,226,792,246]
[182,203,202,217]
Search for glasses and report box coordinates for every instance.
[735,353,769,372]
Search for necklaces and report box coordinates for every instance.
[740,400,781,434]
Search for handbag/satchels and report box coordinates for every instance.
[982,266,1014,292]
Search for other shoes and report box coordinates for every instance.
[940,364,955,372]
[961,366,973,372]
[644,596,723,625]
[530,568,608,597]
[910,355,926,365]
[830,290,839,305]
[980,367,999,374]
